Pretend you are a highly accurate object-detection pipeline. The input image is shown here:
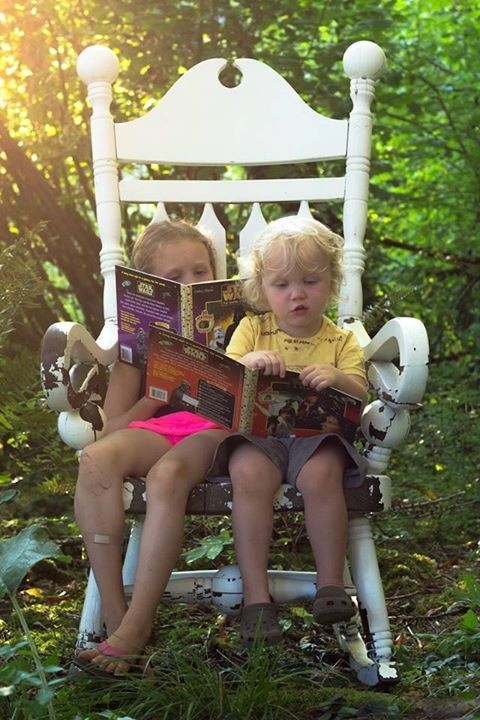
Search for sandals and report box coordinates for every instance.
[240,593,283,647]
[312,585,357,625]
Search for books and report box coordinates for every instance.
[146,323,364,439]
[115,265,251,372]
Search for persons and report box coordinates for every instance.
[222,214,377,643]
[74,218,243,679]
[265,390,349,439]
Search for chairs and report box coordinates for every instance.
[41,38,429,691]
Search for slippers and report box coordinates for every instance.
[73,640,137,682]
